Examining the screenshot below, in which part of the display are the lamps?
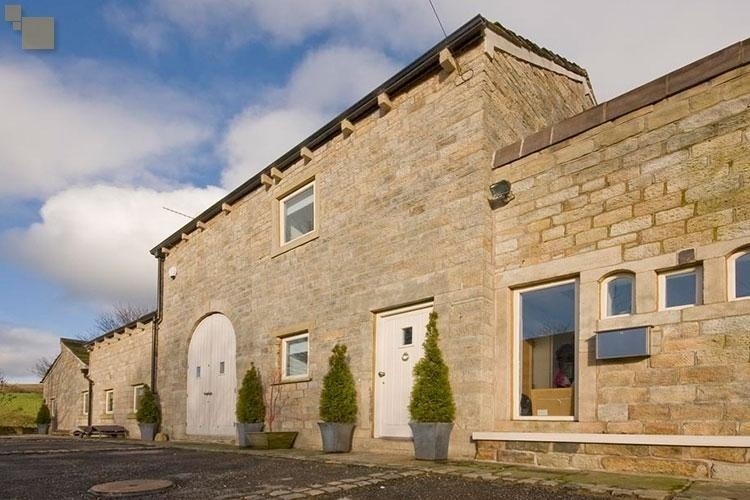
[490,180,515,204]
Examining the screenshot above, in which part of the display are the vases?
[245,431,299,449]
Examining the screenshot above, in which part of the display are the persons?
[551,361,572,388]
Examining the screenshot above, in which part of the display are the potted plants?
[135,384,160,442]
[235,362,266,448]
[34,399,52,435]
[317,343,358,452]
[409,311,457,460]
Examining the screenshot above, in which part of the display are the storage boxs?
[531,388,574,416]
